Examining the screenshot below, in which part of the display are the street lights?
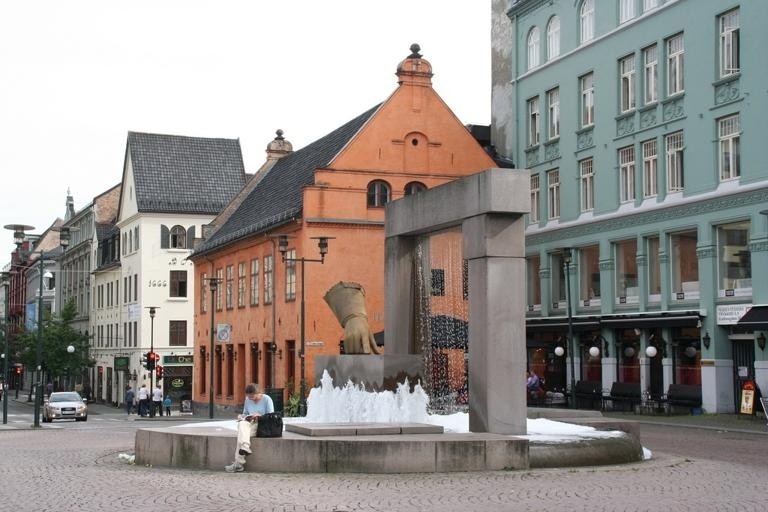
[553,245,583,410]
[4,222,80,426]
[268,232,338,417]
[1,353,7,393]
[145,305,161,416]
[203,277,226,419]
[0,270,20,426]
[13,361,24,398]
[36,363,46,407]
[66,342,75,392]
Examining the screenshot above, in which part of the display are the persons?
[46,381,92,402]
[0,379,5,401]
[528,368,540,388]
[134,387,141,416]
[527,371,531,385]
[222,381,275,473]
[125,387,135,415]
[140,384,149,417]
[322,281,382,354]
[151,385,163,417]
[163,395,171,417]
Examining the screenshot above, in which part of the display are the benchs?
[565,379,702,416]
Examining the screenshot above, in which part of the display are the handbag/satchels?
[257,413,283,437]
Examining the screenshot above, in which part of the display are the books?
[236,415,254,422]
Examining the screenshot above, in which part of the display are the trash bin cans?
[264,388,285,417]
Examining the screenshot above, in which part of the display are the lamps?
[702,332,711,349]
[553,334,609,357]
[757,332,766,351]
[645,336,667,359]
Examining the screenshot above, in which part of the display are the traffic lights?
[21,368,25,375]
[148,353,155,371]
[161,367,166,378]
[142,352,150,370]
[155,354,160,369]
[16,367,21,374]
[156,365,161,379]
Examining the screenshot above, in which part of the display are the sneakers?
[224,463,244,472]
[239,446,252,456]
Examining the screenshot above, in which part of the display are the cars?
[42,391,89,423]
